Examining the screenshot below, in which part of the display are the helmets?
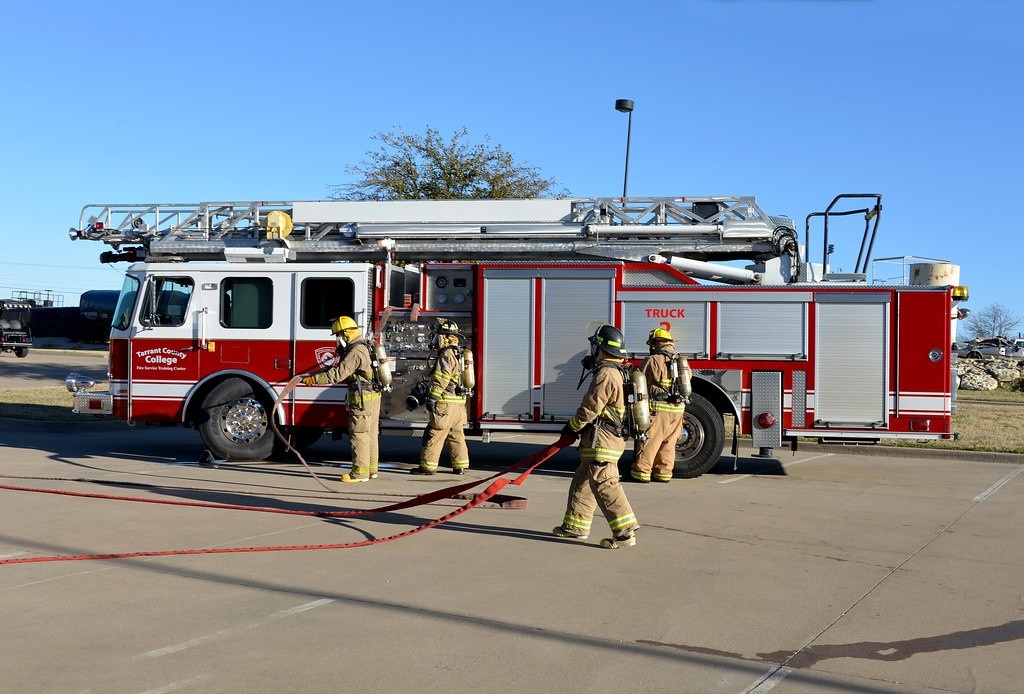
[646,329,676,346]
[590,325,626,358]
[434,321,466,340]
[330,316,358,336]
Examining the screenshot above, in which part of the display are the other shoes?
[341,474,370,483]
[599,527,635,549]
[410,465,436,475]
[650,473,670,483]
[553,527,588,539]
[621,473,649,483]
[452,468,464,475]
[369,474,378,479]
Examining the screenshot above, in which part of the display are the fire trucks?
[66,194,971,479]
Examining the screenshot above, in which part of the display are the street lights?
[615,99,634,226]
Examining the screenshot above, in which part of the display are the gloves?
[426,398,437,413]
[560,423,579,441]
[301,376,318,387]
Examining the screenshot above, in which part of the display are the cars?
[952,337,1024,359]
[0,310,33,358]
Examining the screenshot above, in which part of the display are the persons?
[405,318,469,475]
[553,320,640,549]
[302,316,382,483]
[621,328,693,483]
